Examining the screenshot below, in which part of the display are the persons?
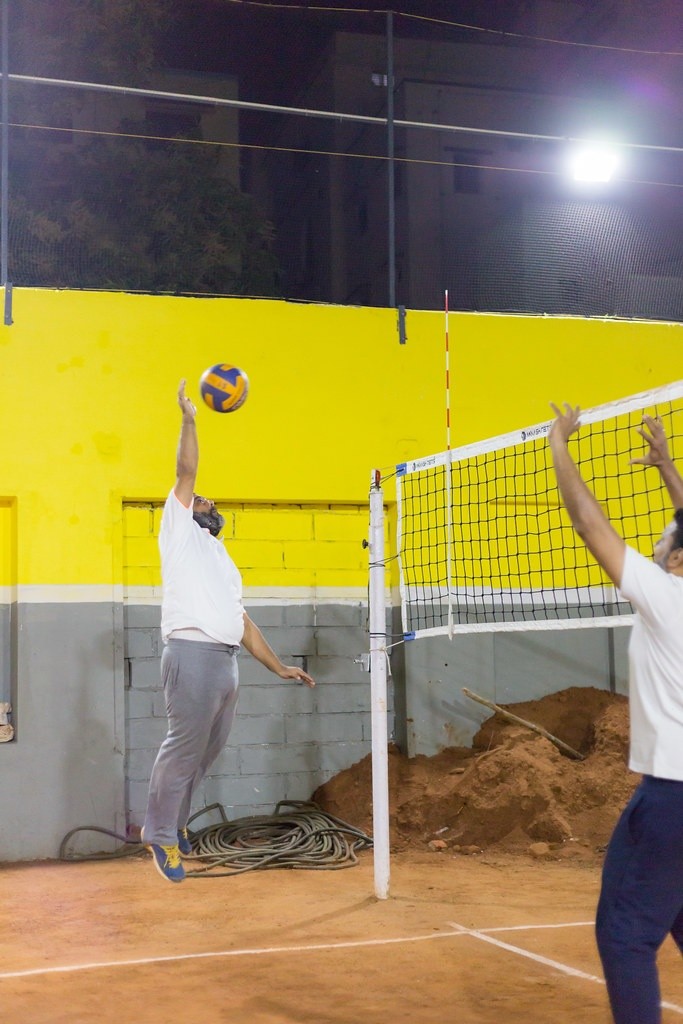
[139,379,318,884]
[548,400,683,1024]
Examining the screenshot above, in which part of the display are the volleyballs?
[200,363,250,413]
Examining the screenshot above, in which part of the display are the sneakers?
[141,826,185,883]
[176,827,192,857]
